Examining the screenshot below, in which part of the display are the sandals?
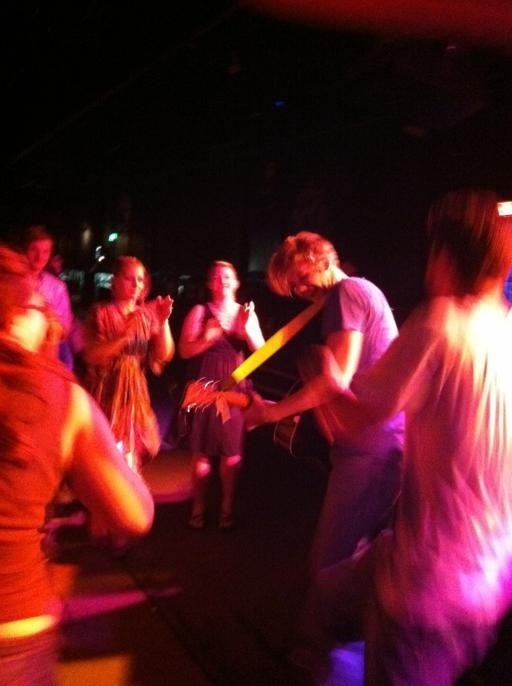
[217,510,233,528]
[188,511,206,529]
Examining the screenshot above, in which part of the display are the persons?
[0,248,156,686]
[240,230,407,673]
[307,197,510,686]
[0,225,88,378]
[81,256,176,549]
[178,260,267,530]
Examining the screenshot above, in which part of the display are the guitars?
[180,376,330,465]
